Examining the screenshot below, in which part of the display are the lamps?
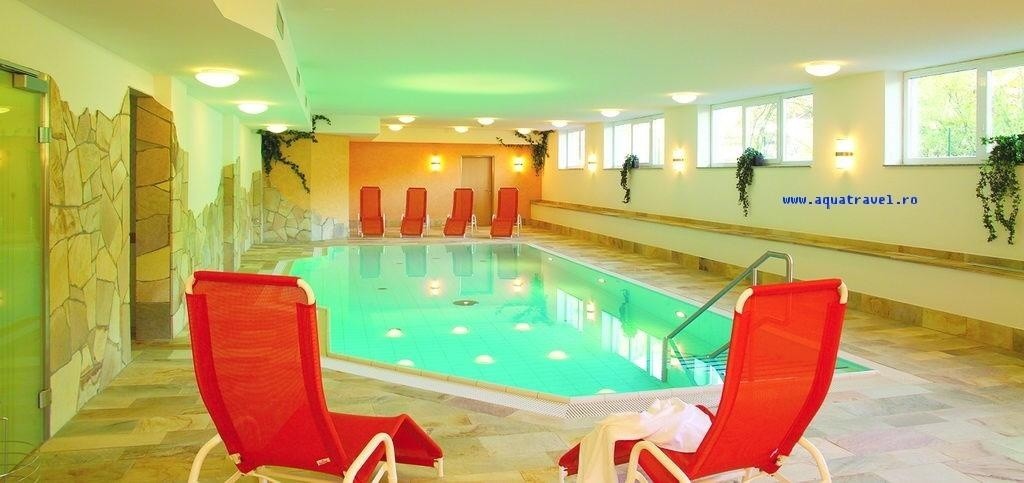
[833,134,857,169]
[512,157,523,173]
[587,153,596,171]
[671,146,686,172]
[428,156,440,172]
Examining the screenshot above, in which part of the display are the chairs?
[552,277,849,483]
[356,183,386,238]
[486,185,522,239]
[441,187,478,237]
[357,244,521,285]
[396,187,431,238]
[182,269,446,483]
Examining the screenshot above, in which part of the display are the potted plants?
[617,153,640,205]
[733,145,766,221]
